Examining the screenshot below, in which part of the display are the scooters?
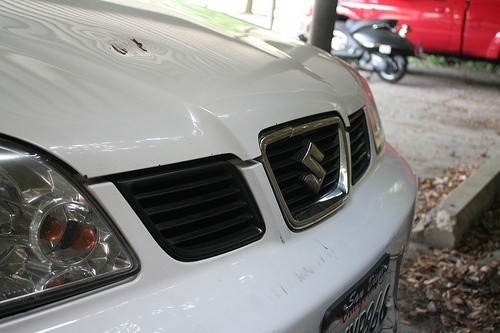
[301,15,414,82]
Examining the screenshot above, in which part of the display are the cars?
[305,0,500,82]
[0,1,416,333]
